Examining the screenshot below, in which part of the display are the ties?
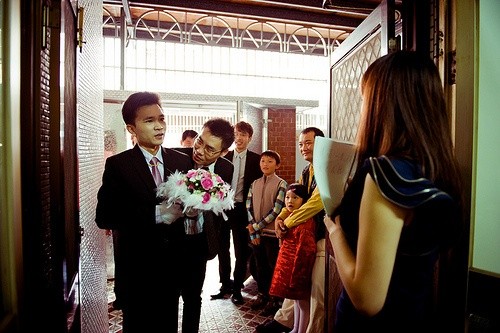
[230,155,241,194]
[150,158,166,189]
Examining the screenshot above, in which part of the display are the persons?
[94,92,201,333]
[180,129,199,149]
[255,126,327,333]
[323,49,462,333]
[170,118,234,333]
[245,150,289,317]
[268,183,317,333]
[210,121,265,305]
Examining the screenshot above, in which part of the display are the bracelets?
[328,228,343,239]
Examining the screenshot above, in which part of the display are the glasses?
[192,129,223,156]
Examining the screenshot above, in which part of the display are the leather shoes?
[232,292,243,304]
[211,287,231,299]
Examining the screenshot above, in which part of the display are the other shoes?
[263,301,282,313]
[248,293,268,308]
[255,319,291,333]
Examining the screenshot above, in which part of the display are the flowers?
[156,168,236,222]
